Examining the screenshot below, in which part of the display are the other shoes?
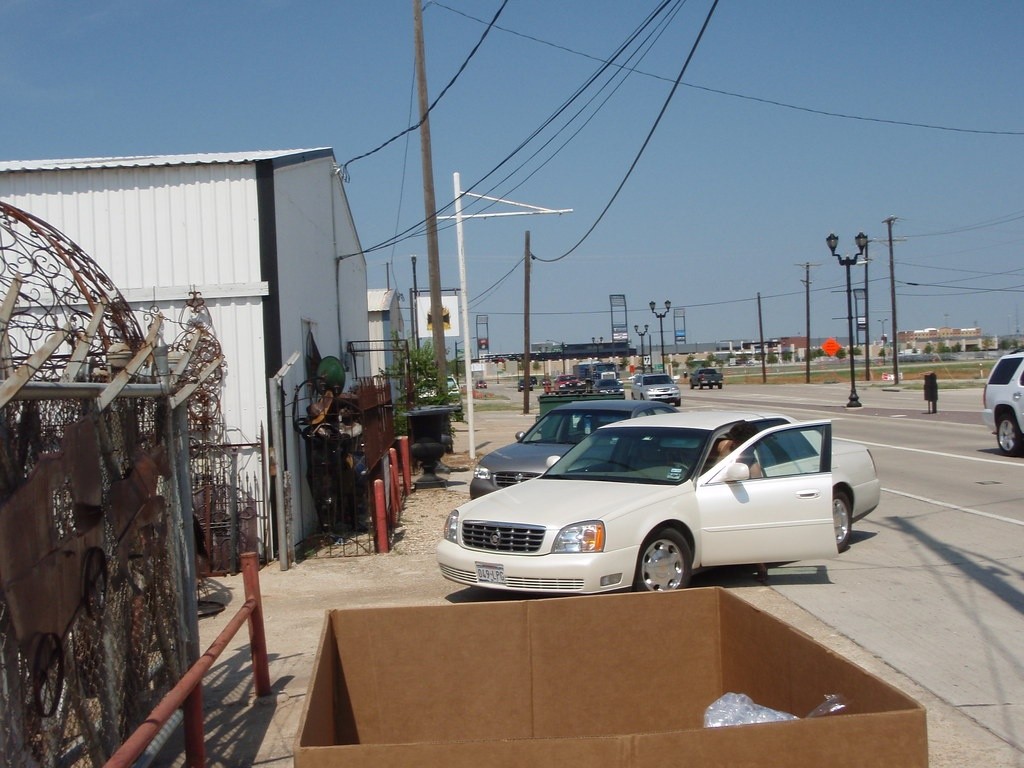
[756,567,768,582]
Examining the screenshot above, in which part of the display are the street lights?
[649,300,672,373]
[634,324,649,374]
[825,231,868,408]
[877,318,888,365]
[592,337,603,361]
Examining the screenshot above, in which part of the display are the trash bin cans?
[923,373,938,414]
[535,392,625,439]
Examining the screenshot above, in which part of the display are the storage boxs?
[292,587,928,768]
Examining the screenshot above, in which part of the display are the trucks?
[572,362,622,382]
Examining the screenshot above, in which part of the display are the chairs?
[643,448,685,471]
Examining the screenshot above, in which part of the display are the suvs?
[630,372,682,407]
[983,349,1024,457]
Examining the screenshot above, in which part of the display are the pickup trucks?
[689,368,724,389]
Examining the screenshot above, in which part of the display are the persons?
[705,423,765,582]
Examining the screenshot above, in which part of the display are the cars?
[475,380,487,390]
[539,379,551,388]
[469,399,681,500]
[530,377,537,386]
[554,371,625,395]
[417,376,460,397]
[436,409,880,595]
[518,379,534,392]
